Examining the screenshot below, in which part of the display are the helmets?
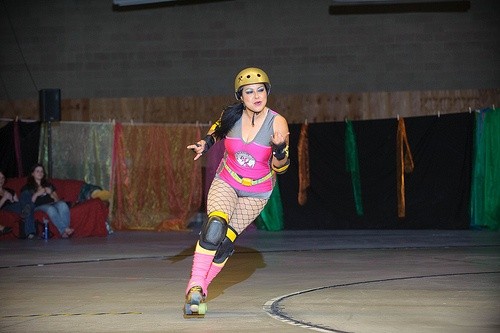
[234,67,271,101]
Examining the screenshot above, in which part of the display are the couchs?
[0,177,109,242]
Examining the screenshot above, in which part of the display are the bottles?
[105,222,113,234]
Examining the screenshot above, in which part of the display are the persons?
[184,66,291,315]
[0,171,37,239]
[21,163,74,238]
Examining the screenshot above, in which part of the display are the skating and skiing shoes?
[183,282,207,318]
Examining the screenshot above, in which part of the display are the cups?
[42,222,49,240]
